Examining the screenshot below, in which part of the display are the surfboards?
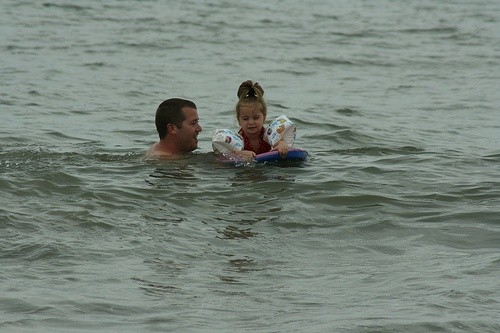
[214,147,309,164]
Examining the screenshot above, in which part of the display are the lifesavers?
[262,115,296,150]
[211,128,245,158]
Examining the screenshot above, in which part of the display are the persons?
[233,79,287,162]
[143,99,202,157]
